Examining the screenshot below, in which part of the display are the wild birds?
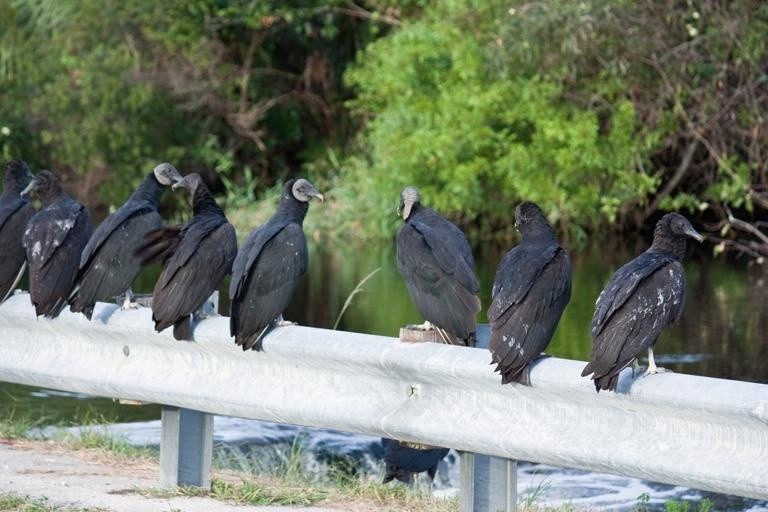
[227,176,326,353]
[18,168,94,322]
[0,157,38,307]
[378,434,451,489]
[132,169,238,343]
[64,159,186,322]
[393,186,485,347]
[580,208,709,393]
[483,199,574,386]
